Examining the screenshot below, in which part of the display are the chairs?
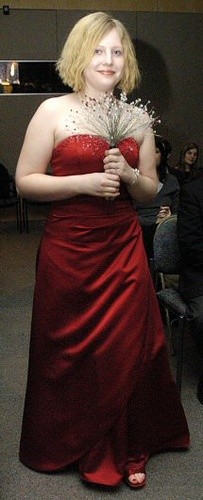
[0,163,22,234]
[153,214,195,400]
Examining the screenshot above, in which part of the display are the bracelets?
[127,168,140,186]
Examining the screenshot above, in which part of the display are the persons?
[15,12,203,490]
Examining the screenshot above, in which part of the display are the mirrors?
[0,59,75,95]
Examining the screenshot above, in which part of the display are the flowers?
[66,91,162,202]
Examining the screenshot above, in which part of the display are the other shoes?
[124,467,146,489]
